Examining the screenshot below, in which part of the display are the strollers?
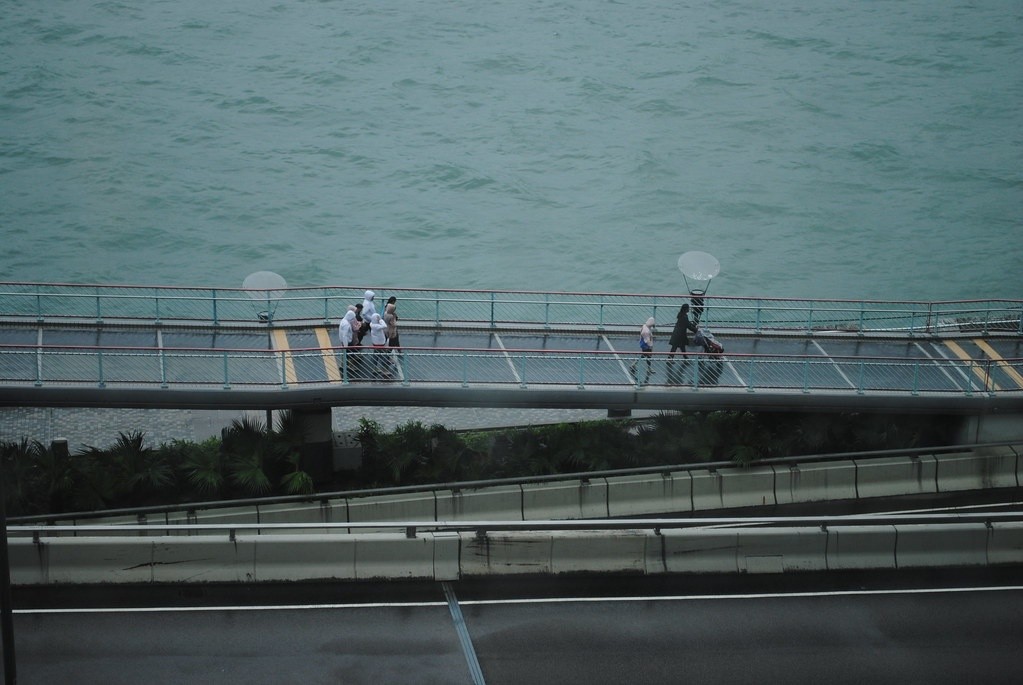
[693,322,724,370]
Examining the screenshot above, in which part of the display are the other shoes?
[382,371,392,376]
[668,360,675,363]
[684,360,695,364]
[630,366,638,371]
[646,369,656,373]
[373,369,383,373]
[348,366,358,371]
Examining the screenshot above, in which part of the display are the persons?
[666,304,698,364]
[338,304,368,373]
[370,313,392,377]
[630,317,656,374]
[381,296,403,358]
[360,290,377,333]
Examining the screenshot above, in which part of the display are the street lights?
[677,251,721,328]
[241,271,288,433]
